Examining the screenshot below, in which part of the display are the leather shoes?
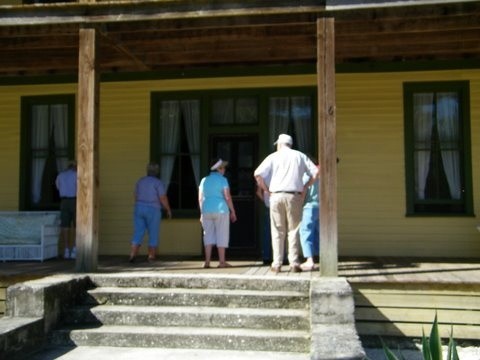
[271,264,303,273]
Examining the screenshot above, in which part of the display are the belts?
[275,190,301,194]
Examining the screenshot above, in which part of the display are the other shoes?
[123,254,158,263]
[64,247,76,259]
[300,262,314,268]
[201,259,233,269]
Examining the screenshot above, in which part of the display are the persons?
[129,163,172,258]
[198,159,238,269]
[256,182,273,265]
[55,160,79,258]
[253,133,319,272]
[299,153,319,269]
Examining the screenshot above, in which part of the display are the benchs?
[0,210,62,263]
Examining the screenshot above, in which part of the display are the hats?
[274,133,293,146]
[210,159,228,171]
[64,161,76,169]
